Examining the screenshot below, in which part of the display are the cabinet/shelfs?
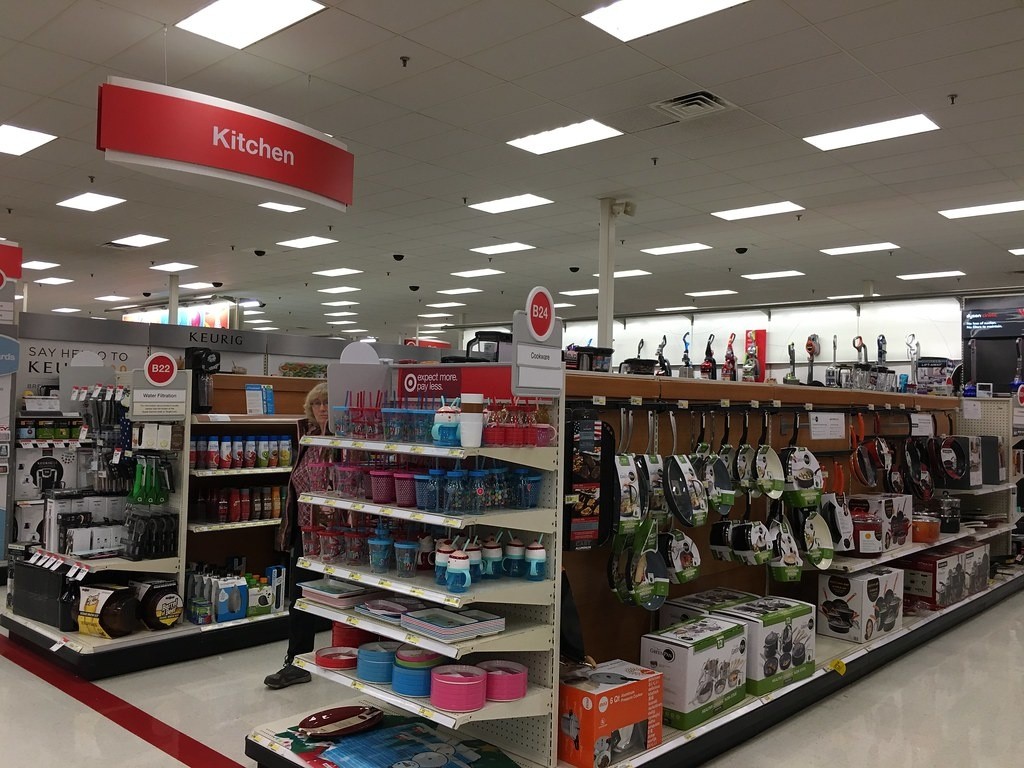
[0,372,130,586]
[0,373,329,683]
[245,360,1024,768]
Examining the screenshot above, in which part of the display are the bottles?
[189,434,294,522]
[242,573,270,589]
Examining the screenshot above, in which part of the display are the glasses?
[311,401,328,406]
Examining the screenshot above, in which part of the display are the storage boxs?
[640,586,816,730]
[14,418,127,557]
[558,660,664,768]
[818,567,903,643]
[844,492,913,553]
[882,541,990,611]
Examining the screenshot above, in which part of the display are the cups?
[300,405,558,592]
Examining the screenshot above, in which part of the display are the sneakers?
[264,655,311,689]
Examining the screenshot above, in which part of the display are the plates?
[296,579,506,643]
[298,706,383,736]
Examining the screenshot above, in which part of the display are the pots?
[608,411,1024,609]
[761,624,811,677]
[682,592,789,614]
[687,655,748,705]
[560,568,597,670]
[820,500,992,633]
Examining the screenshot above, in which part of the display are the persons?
[263,381,340,690]
